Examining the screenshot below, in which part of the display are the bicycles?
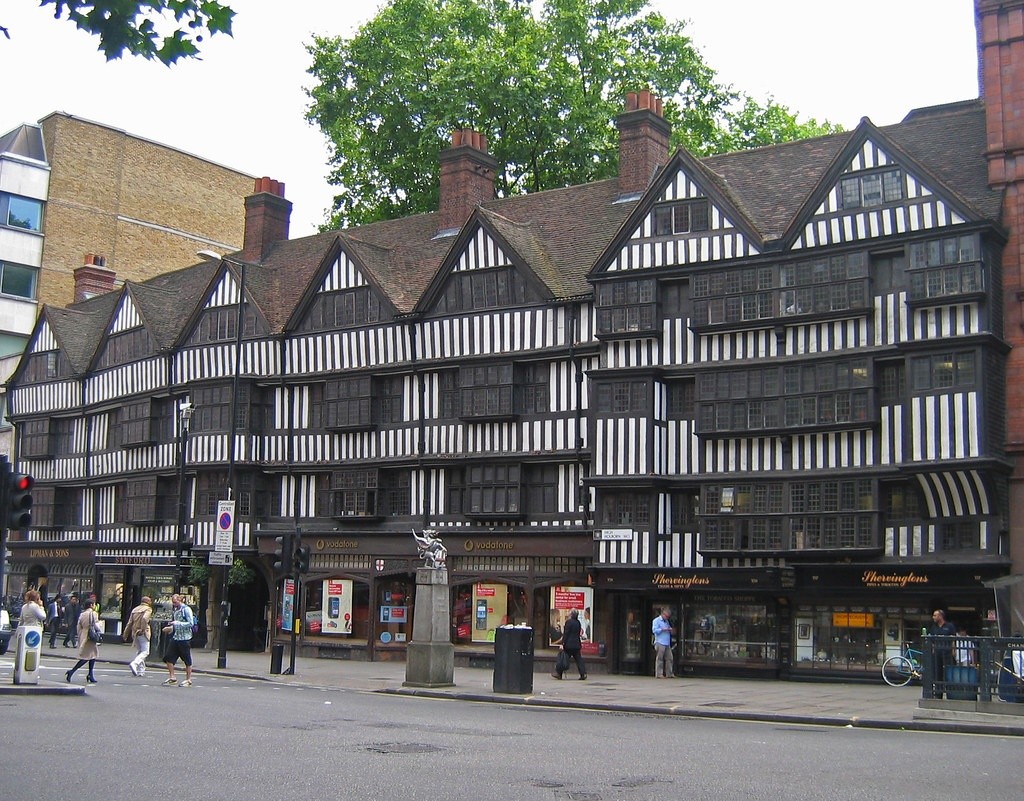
[881,640,925,688]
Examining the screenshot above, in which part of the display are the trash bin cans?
[144,620,170,662]
[492,627,535,694]
[270,644,284,674]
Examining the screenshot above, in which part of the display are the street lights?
[194,247,248,670]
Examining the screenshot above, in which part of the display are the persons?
[65,598,100,682]
[162,593,194,687]
[651,606,678,679]
[16,590,46,637]
[928,609,956,700]
[953,627,976,667]
[46,594,102,649]
[123,596,152,677]
[551,608,587,680]
[1004,632,1024,684]
[550,609,564,646]
[583,607,590,640]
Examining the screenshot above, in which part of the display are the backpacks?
[181,605,198,633]
[556,650,569,673]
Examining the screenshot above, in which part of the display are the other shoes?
[73,645,78,649]
[50,644,57,649]
[130,662,138,677]
[139,672,145,676]
[551,672,562,680]
[667,675,677,679]
[63,642,70,648]
[656,675,665,679]
[162,677,178,686]
[578,672,587,681]
[179,680,192,687]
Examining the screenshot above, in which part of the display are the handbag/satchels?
[89,612,100,642]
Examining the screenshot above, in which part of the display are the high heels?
[65,670,74,683]
[87,674,98,683]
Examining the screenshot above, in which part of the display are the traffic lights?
[293,547,309,574]
[4,471,36,531]
[274,534,296,573]
